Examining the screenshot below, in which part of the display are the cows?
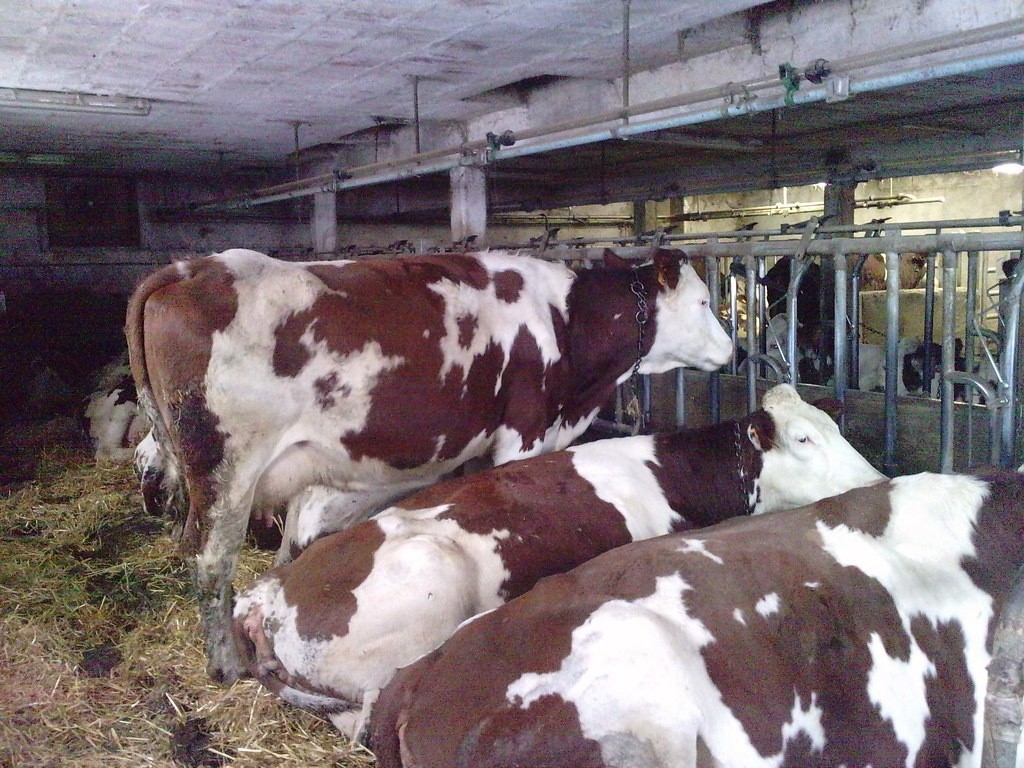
[77,250,1024,768]
